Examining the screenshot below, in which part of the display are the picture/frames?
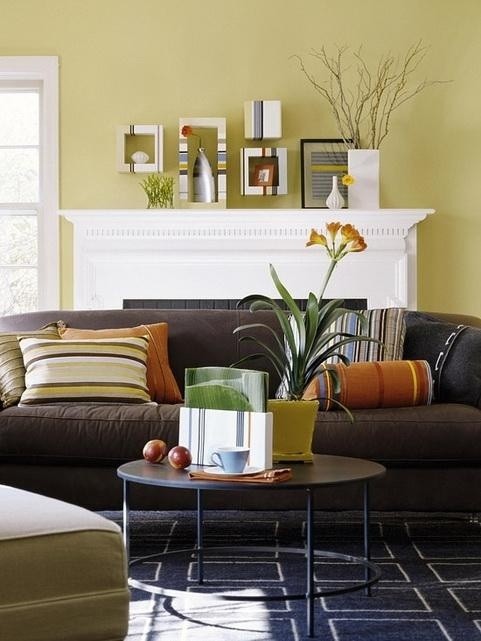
[300,137,358,209]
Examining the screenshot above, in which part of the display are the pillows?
[0,321,67,409]
[403,311,481,408]
[274,307,410,401]
[16,335,151,404]
[56,320,185,405]
[301,359,434,409]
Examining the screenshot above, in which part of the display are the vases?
[347,148,381,211]
[261,397,322,464]
[325,176,346,209]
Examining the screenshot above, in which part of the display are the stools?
[0,482,131,641]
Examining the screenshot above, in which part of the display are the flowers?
[228,217,387,426]
[335,171,357,185]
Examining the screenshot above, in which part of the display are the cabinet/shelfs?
[113,100,289,207]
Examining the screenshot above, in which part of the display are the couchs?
[0,307,481,514]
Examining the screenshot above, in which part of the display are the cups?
[210,447,251,473]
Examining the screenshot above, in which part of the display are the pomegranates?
[142,439,167,464]
[167,447,192,469]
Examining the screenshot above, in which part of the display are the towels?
[186,465,294,485]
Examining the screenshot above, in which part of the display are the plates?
[203,466,266,476]
